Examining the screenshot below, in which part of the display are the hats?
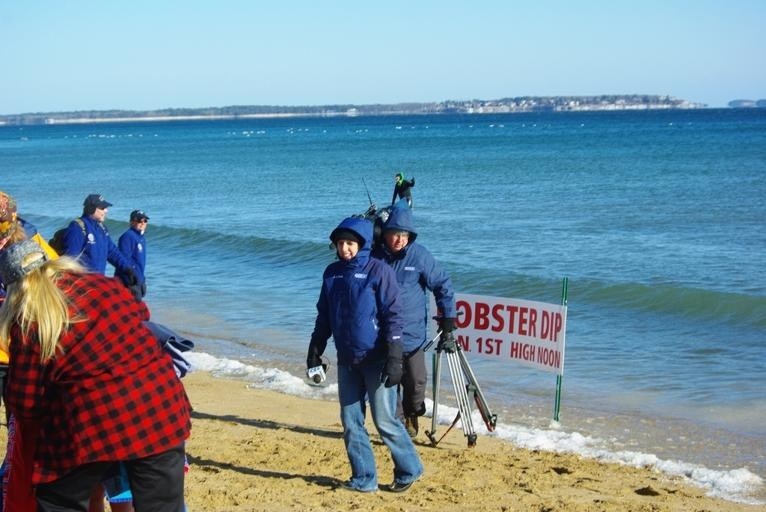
[332,232,361,244]
[0,191,17,243]
[0,240,48,291]
[84,195,112,210]
[131,211,150,220]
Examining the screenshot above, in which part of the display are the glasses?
[137,220,147,223]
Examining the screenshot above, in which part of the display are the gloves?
[438,317,457,333]
[381,358,403,387]
[307,354,327,375]
[126,270,137,286]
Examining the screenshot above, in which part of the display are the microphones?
[306,363,328,386]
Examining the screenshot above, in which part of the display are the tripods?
[423,316,498,448]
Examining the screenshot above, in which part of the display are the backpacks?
[49,218,86,260]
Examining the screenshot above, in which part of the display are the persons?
[0,241,193,512]
[1,190,59,432]
[369,199,456,438]
[306,219,424,492]
[391,172,415,209]
[62,193,138,287]
[88,473,134,512]
[114,210,148,302]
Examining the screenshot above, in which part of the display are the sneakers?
[387,479,412,491]
[406,416,418,437]
[340,481,356,490]
